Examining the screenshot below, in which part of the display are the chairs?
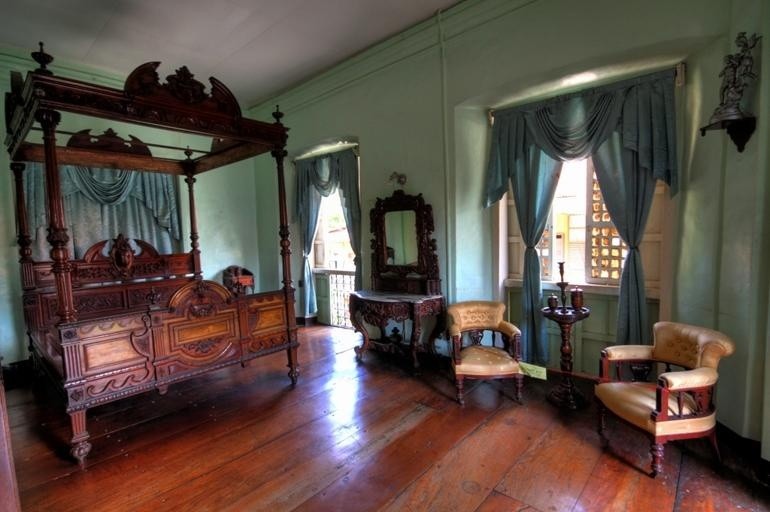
[443,300,525,408]
[595,320,737,477]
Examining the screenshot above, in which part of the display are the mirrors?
[371,188,439,281]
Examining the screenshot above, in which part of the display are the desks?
[542,307,590,412]
[349,289,444,368]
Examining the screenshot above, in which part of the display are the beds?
[27,235,300,469]
